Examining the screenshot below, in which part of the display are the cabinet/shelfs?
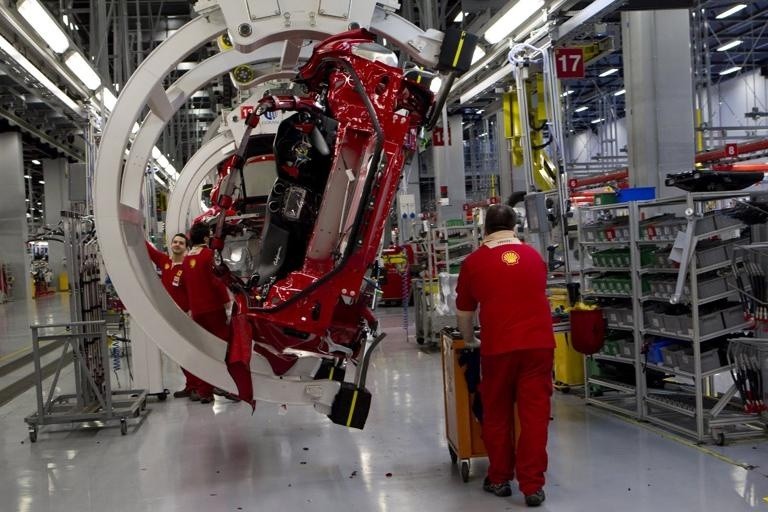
[432,215,479,280]
[577,191,761,445]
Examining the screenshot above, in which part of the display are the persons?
[145,223,242,403]
[454,203,556,507]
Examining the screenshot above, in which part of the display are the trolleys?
[434,319,527,484]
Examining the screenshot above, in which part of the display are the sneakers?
[482,473,512,497]
[173,387,215,404]
[523,486,545,508]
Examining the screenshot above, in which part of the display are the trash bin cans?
[552,321,591,393]
